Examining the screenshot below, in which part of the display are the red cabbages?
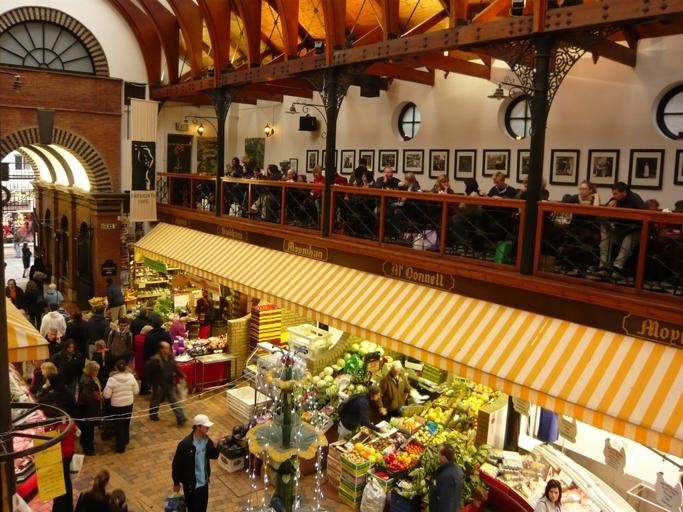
[218,408,272,450]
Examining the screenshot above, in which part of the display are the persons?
[4,213,34,278]
[429,445,464,512]
[4,259,232,455]
[171,414,223,512]
[337,386,385,440]
[373,362,415,424]
[271,459,296,512]
[533,479,562,512]
[73,470,129,512]
[194,156,683,295]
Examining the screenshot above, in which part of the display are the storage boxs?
[326,360,493,512]
[218,444,245,473]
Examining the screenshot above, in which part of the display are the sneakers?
[150,416,160,421]
[177,418,188,426]
[83,451,95,456]
[116,446,124,452]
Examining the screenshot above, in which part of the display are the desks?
[192,352,240,400]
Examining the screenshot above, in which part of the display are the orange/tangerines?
[404,443,425,455]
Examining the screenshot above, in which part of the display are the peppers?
[384,452,418,472]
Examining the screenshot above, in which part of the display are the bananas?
[432,430,458,445]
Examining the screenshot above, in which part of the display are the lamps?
[487,83,534,135]
[184,115,217,132]
[265,124,274,138]
[286,102,328,138]
[0,71,21,92]
[197,124,204,136]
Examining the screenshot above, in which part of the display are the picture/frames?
[289,149,531,183]
[549,148,683,191]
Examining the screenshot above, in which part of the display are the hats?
[193,414,214,427]
[95,340,109,352]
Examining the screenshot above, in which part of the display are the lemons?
[462,383,502,416]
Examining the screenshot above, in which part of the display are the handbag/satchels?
[163,492,186,511]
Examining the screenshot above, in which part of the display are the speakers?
[299,116,316,130]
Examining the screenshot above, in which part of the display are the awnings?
[134,222,683,458]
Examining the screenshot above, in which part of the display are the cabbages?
[311,340,385,390]
